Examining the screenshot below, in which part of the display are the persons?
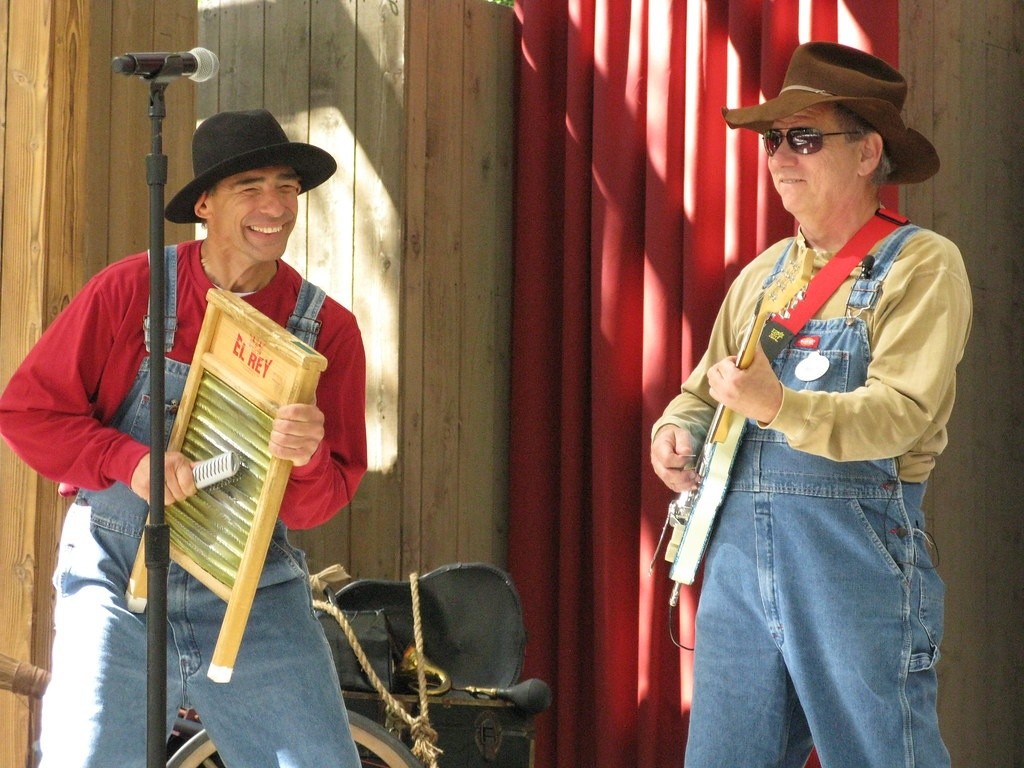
[1,108,367,768]
[651,40,977,768]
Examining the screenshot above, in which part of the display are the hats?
[721,41,940,186]
[164,108,338,224]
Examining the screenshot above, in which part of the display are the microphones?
[113,47,219,83]
[862,255,875,274]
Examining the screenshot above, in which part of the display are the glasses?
[762,127,869,155]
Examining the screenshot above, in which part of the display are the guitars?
[661,244,817,586]
